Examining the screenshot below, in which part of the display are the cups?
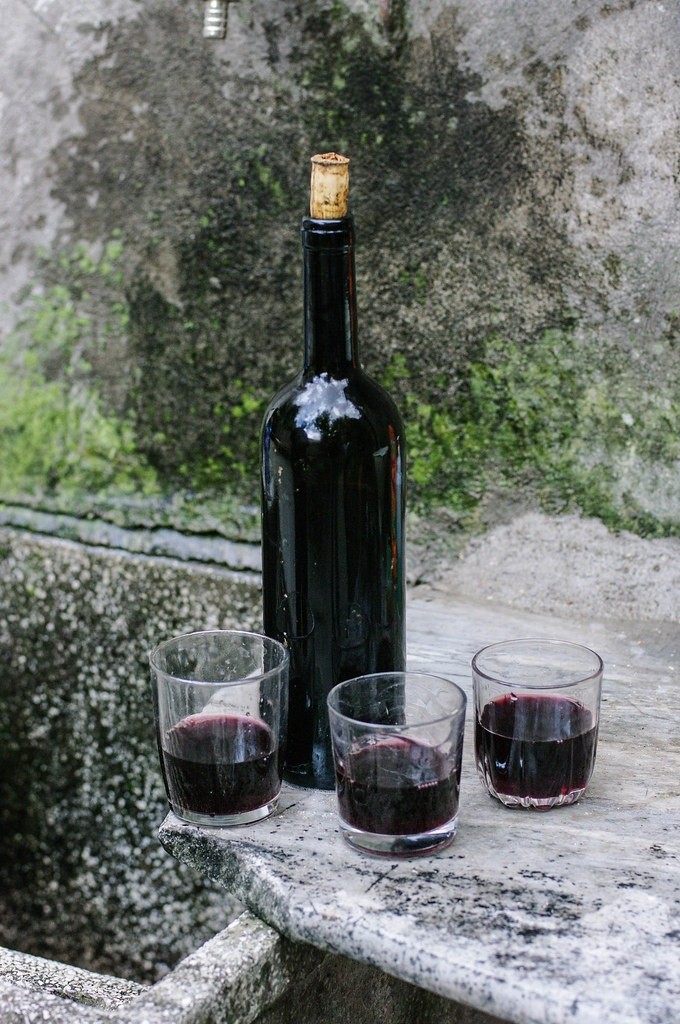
[146,630,289,827]
[471,641,604,811]
[325,671,467,859]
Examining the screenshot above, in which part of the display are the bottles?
[260,153,407,789]
